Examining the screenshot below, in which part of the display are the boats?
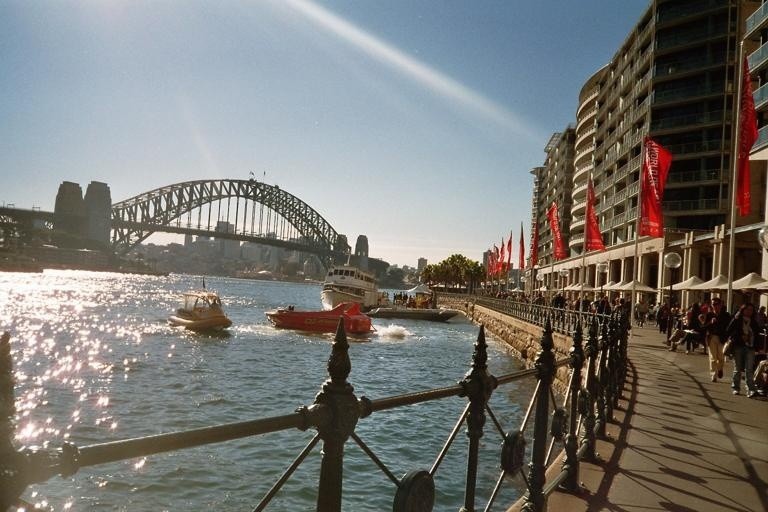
[264,301,377,334]
[321,254,393,312]
[168,276,233,334]
[364,305,459,322]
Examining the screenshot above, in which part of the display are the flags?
[485,170,605,340]
[635,133,673,239]
[733,44,760,215]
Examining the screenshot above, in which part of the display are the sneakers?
[732,390,739,395]
[747,392,756,398]
[717,369,723,379]
[712,375,717,382]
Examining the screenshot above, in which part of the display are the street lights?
[486,281,491,296]
[758,225,768,255]
[492,280,499,297]
[536,273,544,305]
[500,279,506,298]
[596,261,609,313]
[465,281,468,294]
[508,277,514,299]
[663,252,682,346]
[558,267,570,309]
[481,282,485,296]
[520,276,527,301]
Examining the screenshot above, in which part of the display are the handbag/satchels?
[722,340,733,355]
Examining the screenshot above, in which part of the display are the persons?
[391,292,438,310]
[210,298,219,309]
[287,305,294,313]
[481,271,767,396]
